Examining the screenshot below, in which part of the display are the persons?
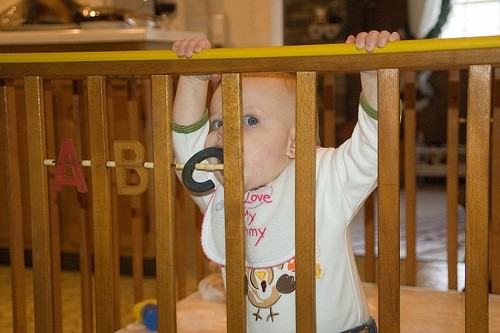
[171,30,401,333]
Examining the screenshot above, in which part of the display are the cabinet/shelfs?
[0,29,208,261]
